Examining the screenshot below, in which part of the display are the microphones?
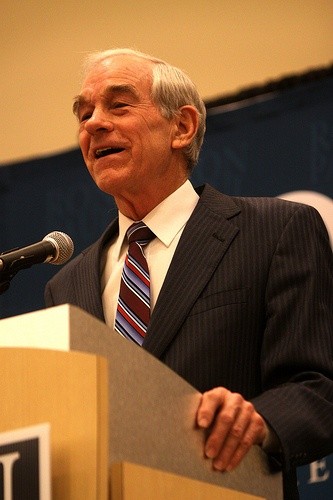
[0,231,74,273]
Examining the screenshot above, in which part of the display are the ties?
[113,221,157,346]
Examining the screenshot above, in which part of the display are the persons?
[44,48,333,500]
